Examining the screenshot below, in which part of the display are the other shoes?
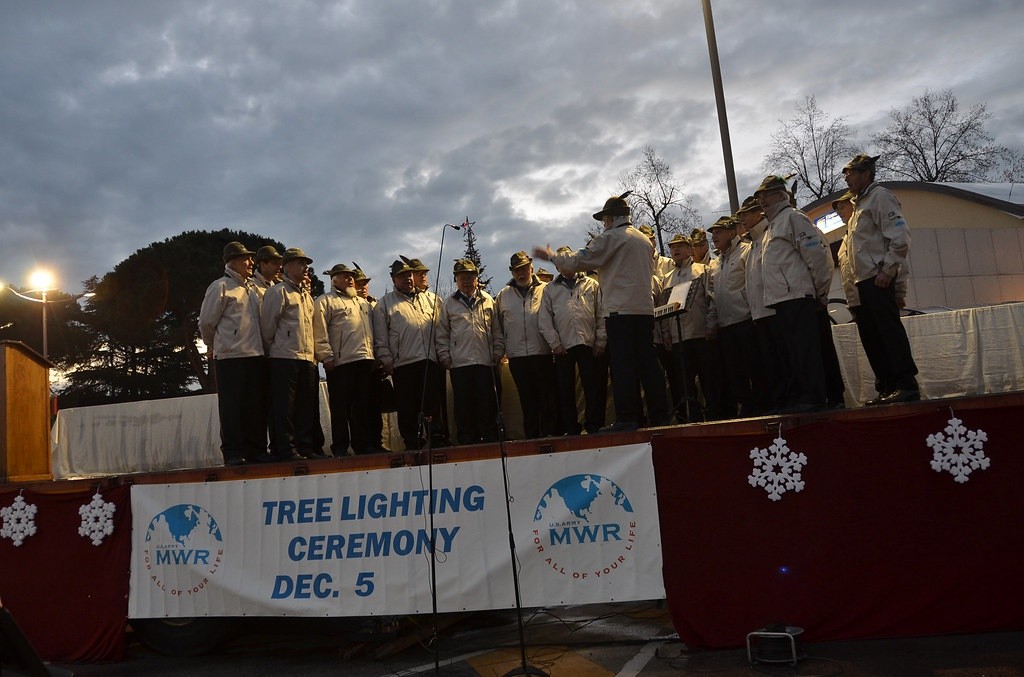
[404,432,506,451]
[226,441,393,463]
[598,419,641,431]
[865,387,920,405]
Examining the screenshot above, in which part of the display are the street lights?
[0,268,95,361]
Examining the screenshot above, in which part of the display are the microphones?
[446,223,460,230]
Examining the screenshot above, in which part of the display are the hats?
[752,174,787,196]
[832,192,855,210]
[736,196,761,215]
[842,155,881,173]
[388,260,414,274]
[668,233,690,245]
[689,229,707,242]
[350,269,372,283]
[509,252,532,269]
[281,248,312,263]
[254,246,283,262]
[327,264,356,275]
[639,225,656,239]
[556,246,573,253]
[405,259,430,272]
[454,259,479,273]
[707,216,733,232]
[535,268,554,279]
[223,242,256,263]
[593,197,631,221]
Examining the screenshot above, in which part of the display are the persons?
[533,196,672,433]
[638,154,920,424]
[200,242,609,464]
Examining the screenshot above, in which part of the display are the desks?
[49,381,331,481]
[830,302,1024,408]
